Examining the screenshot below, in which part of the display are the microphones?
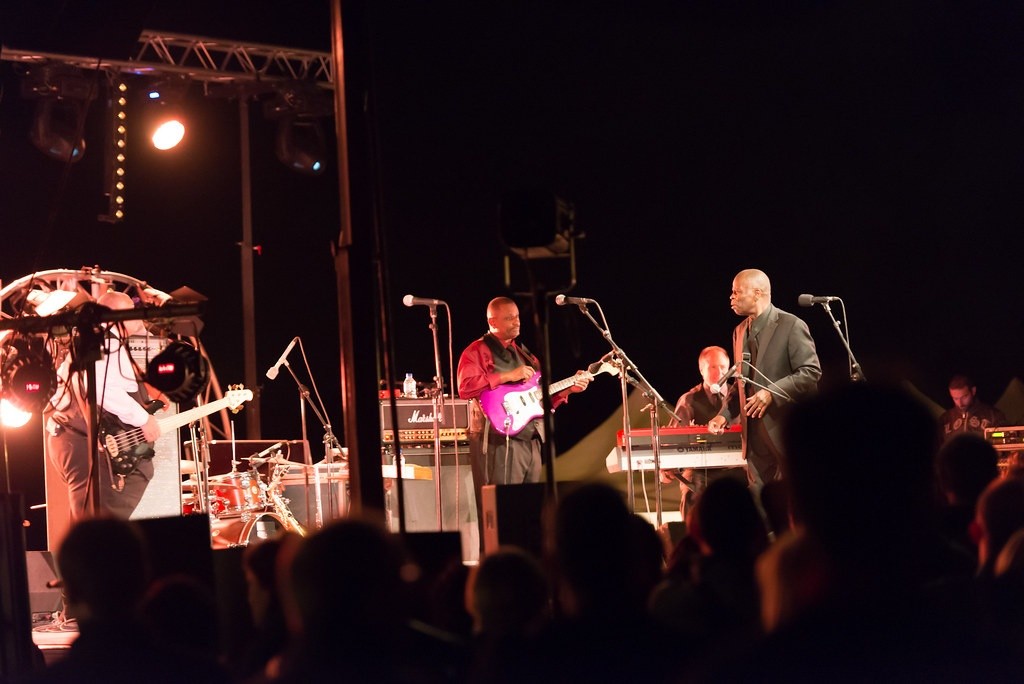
[265,338,297,380]
[588,351,616,374]
[798,294,838,307]
[710,361,741,394]
[403,295,445,307]
[555,294,594,305]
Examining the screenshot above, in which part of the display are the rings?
[757,408,761,410]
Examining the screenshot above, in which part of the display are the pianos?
[606,423,748,531]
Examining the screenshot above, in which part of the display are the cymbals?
[181,459,210,475]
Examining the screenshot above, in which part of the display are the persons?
[935,374,1012,458]
[42,291,161,523]
[457,296,595,556]
[30,378,1024,684]
[659,269,823,542]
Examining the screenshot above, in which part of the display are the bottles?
[393,449,405,466]
[404,373,416,397]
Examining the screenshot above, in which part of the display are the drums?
[210,471,265,513]
[211,511,290,549]
[179,492,214,514]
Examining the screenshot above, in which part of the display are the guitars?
[477,350,623,436]
[97,383,254,477]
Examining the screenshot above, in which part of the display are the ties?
[507,344,522,366]
[715,391,722,411]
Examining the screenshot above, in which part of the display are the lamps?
[143,104,185,150]
